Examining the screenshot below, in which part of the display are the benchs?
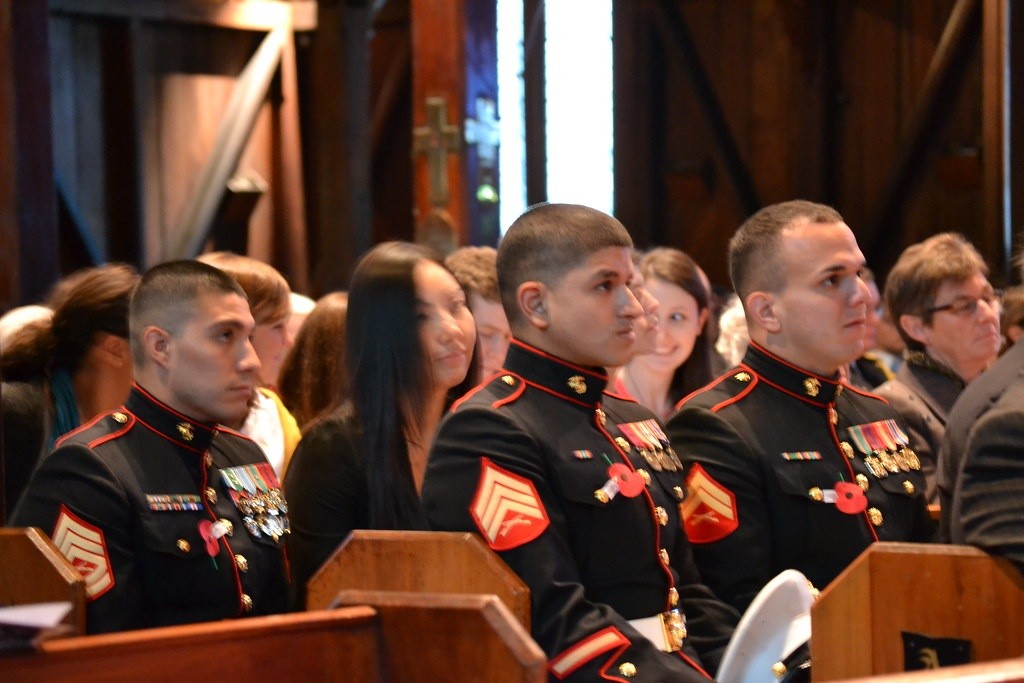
[1,525,86,635]
[0,590,547,683]
[306,530,530,638]
[810,544,1024,683]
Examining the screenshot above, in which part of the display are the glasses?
[912,283,1006,317]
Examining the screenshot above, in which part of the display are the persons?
[0,232,1023,520]
[661,201,971,683]
[937,332,1024,580]
[421,205,741,683]
[0,259,300,656]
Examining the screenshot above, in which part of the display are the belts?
[626,607,687,653]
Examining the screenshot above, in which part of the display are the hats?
[715,568,821,683]
